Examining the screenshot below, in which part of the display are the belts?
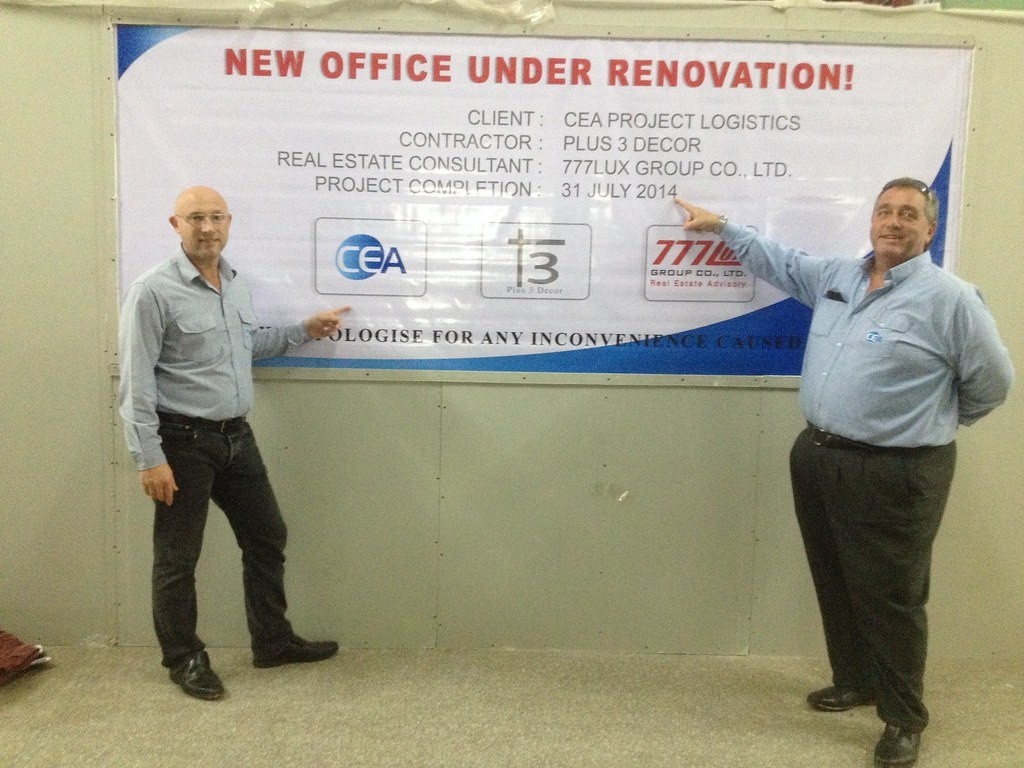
[806,421,921,457]
[156,412,247,433]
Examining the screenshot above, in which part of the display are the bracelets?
[714,214,729,235]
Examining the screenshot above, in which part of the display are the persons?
[672,175,1014,768]
[114,186,354,701]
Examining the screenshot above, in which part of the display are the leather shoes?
[253,634,338,668]
[808,685,875,712]
[170,650,224,699]
[874,721,921,768]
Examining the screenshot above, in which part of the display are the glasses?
[176,213,231,226]
[883,177,932,199]
